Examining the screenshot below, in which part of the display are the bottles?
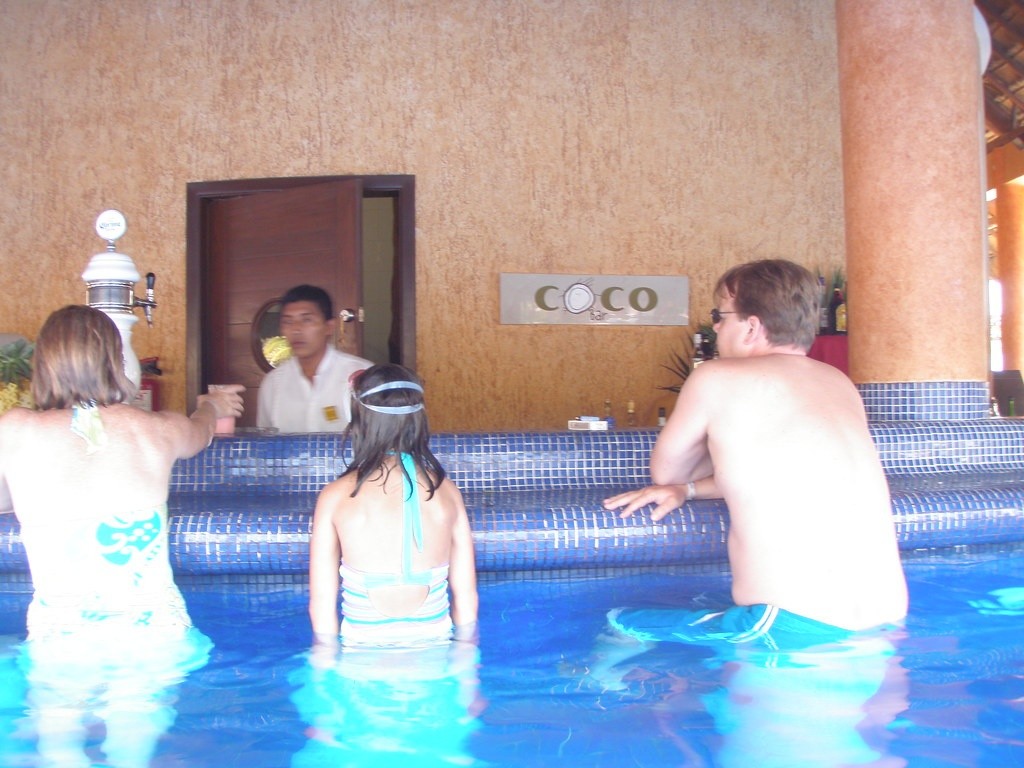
[601,400,615,429]
[625,399,640,426]
[656,408,667,426]
[830,287,847,334]
[1007,397,1015,416]
[690,333,719,368]
[817,277,829,334]
[992,399,999,416]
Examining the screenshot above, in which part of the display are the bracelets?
[687,481,697,502]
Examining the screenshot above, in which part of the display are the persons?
[306,364,481,656]
[600,259,910,660]
[0,303,247,650]
[257,284,375,432]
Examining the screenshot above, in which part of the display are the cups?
[209,384,235,437]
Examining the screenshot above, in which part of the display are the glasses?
[710,309,742,323]
